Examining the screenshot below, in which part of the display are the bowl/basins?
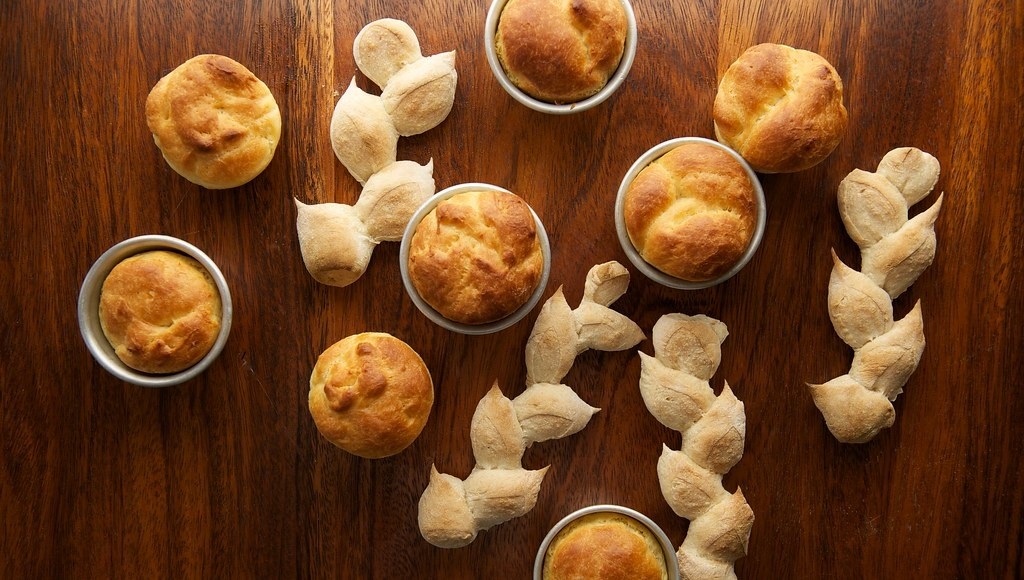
[483,0,636,114]
[614,137,766,287]
[77,234,233,387]
[533,504,680,580]
[400,184,554,335]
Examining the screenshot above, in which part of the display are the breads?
[97,0,946,580]
